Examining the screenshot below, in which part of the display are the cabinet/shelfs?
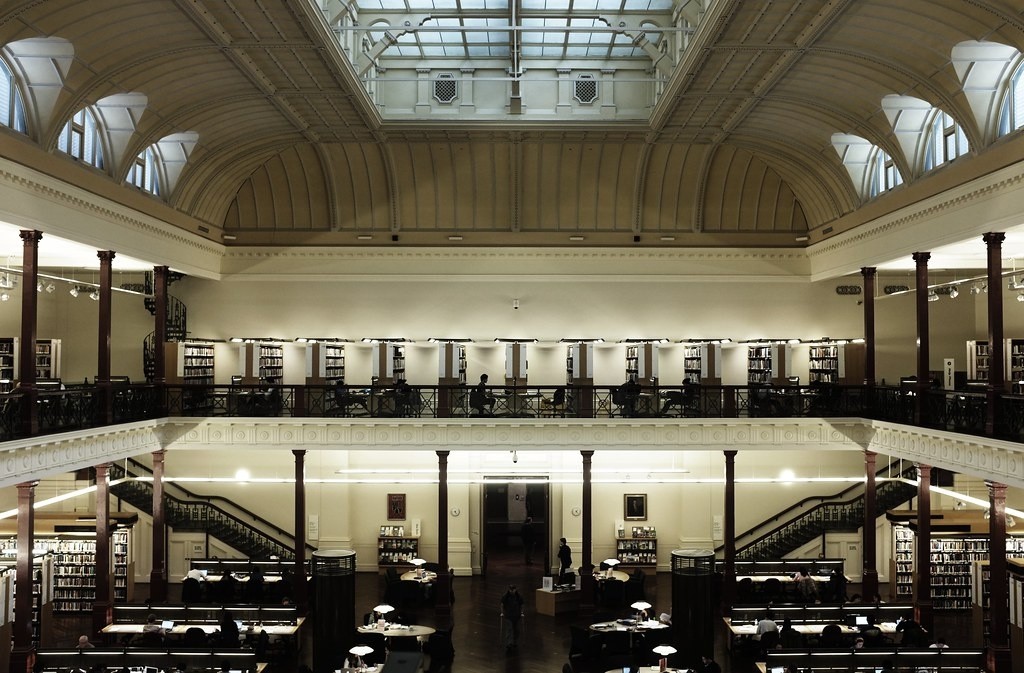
[164,339,868,418]
[616,538,659,575]
[0,337,62,394]
[891,525,1024,610]
[1007,338,1024,382]
[967,339,990,381]
[0,511,139,666]
[374,537,420,576]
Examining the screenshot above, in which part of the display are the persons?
[613,379,635,415]
[520,517,547,566]
[475,373,496,416]
[699,655,721,673]
[661,377,695,414]
[558,537,572,587]
[261,378,281,417]
[501,584,525,650]
[735,567,949,673]
[336,380,367,409]
[75,570,287,673]
[396,379,415,418]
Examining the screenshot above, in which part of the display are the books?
[896,527,1024,610]
[0,533,127,644]
[2,339,1024,406]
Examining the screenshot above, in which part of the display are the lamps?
[0,253,100,301]
[603,559,620,577]
[349,644,374,666]
[653,645,677,673]
[630,601,652,622]
[409,559,427,569]
[372,603,395,619]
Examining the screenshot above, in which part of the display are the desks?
[335,568,687,672]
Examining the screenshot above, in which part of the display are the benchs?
[32,557,328,672]
[718,600,985,673]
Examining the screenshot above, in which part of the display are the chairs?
[490,392,542,417]
[343,391,392,416]
[469,389,487,411]
[779,391,815,418]
[925,258,1024,303]
[543,389,565,412]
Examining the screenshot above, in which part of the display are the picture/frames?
[625,493,648,521]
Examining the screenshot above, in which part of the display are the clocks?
[451,508,461,516]
[572,507,581,516]
